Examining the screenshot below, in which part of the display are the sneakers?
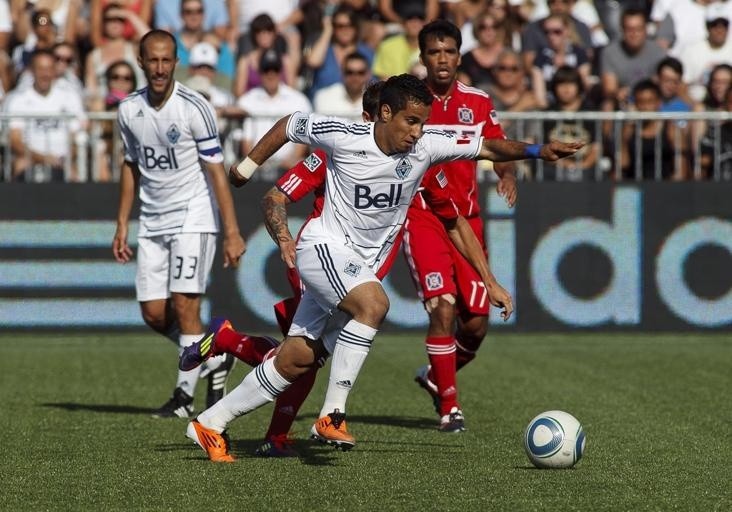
[413,364,466,433]
[153,318,356,461]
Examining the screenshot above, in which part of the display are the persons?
[111,28,249,419]
[184,73,587,462]
[0,0,732,181]
[401,18,518,432]
[176,80,515,458]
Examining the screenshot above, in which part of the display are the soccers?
[524,410,586,468]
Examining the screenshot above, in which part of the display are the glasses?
[183,6,201,15]
[344,67,366,77]
[57,54,72,66]
[335,22,352,30]
[111,74,132,82]
[479,23,563,73]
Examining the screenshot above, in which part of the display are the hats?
[189,42,220,68]
[708,1,730,23]
[258,49,284,68]
